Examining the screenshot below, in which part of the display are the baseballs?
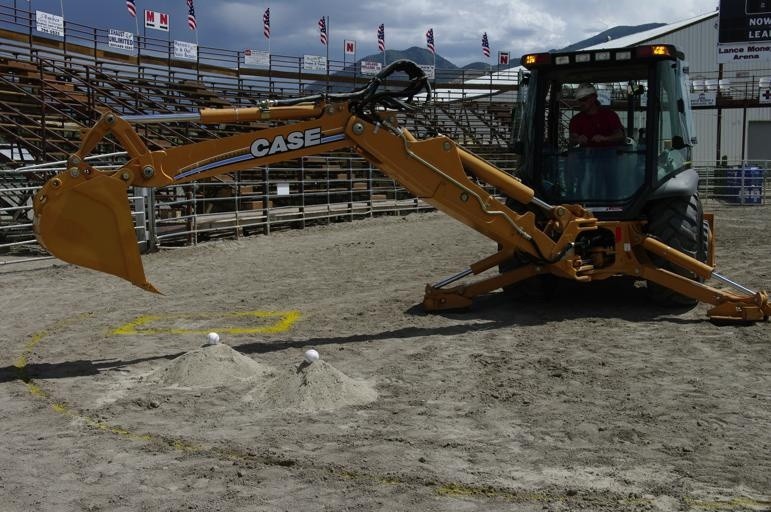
[207,332,219,345]
[304,351,318,366]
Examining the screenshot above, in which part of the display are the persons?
[564,81,627,203]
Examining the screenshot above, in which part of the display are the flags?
[377,23,385,54]
[127,0,137,19]
[263,7,271,40]
[426,28,436,56]
[318,16,328,46]
[481,31,490,58]
[187,0,198,32]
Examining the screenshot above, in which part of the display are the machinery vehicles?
[30,41,771,327]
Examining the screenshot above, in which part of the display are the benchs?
[0,44,517,252]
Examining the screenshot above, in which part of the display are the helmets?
[575,83,596,101]
